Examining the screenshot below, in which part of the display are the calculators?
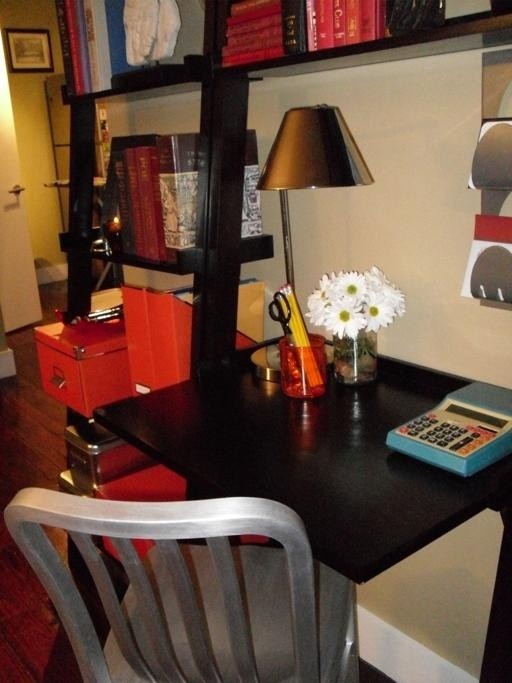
[385,381,512,478]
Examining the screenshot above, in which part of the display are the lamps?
[245,101,380,387]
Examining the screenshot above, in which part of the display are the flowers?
[306,267,411,364]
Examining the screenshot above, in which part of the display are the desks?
[93,333,512,683]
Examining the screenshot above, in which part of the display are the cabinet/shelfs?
[41,71,119,280]
[51,0,239,617]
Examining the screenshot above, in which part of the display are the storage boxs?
[56,468,93,496]
[91,456,274,563]
[33,312,125,418]
[64,420,157,486]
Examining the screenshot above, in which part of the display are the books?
[99,127,263,264]
[51,0,140,98]
[218,0,389,66]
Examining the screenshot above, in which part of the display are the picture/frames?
[4,27,54,74]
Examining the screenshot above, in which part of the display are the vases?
[331,330,377,383]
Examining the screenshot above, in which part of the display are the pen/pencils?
[277,283,324,387]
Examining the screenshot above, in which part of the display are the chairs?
[4,486,360,683]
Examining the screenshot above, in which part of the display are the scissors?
[269,292,295,346]
